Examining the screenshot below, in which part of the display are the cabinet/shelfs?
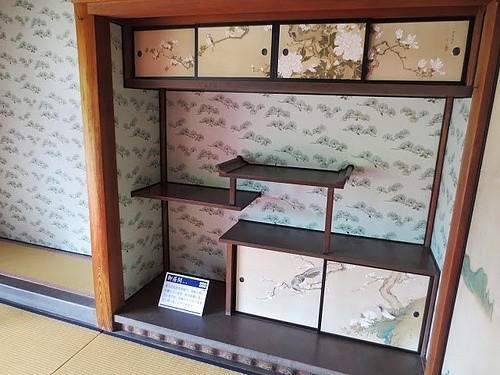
[109,6,486,374]
